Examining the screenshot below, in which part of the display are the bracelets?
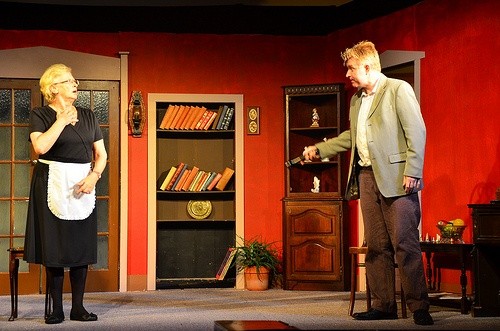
[93,170,101,178]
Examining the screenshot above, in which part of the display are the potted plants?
[231,232,280,291]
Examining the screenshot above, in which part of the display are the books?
[160,163,234,191]
[216,248,236,280]
[159,105,234,130]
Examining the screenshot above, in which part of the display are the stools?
[6,248,51,322]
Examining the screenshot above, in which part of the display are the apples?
[437,220,447,226]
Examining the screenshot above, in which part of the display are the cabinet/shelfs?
[281,83,347,292]
[467,204,500,317]
[147,93,245,290]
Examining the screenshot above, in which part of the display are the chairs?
[349,237,407,319]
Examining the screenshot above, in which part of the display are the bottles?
[419,233,441,244]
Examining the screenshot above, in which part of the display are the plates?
[187,200,212,220]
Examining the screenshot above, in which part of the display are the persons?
[312,109,319,125]
[300,41,434,325]
[22,63,107,324]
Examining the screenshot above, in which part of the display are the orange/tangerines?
[453,219,464,226]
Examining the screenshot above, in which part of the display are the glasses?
[52,79,80,86]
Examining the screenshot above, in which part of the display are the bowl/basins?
[437,225,466,244]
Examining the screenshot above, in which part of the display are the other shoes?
[70,308,98,321]
[413,310,434,325]
[353,307,399,320]
[45,311,65,324]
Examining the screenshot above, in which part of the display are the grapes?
[444,224,457,231]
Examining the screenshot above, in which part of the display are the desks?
[419,242,473,315]
